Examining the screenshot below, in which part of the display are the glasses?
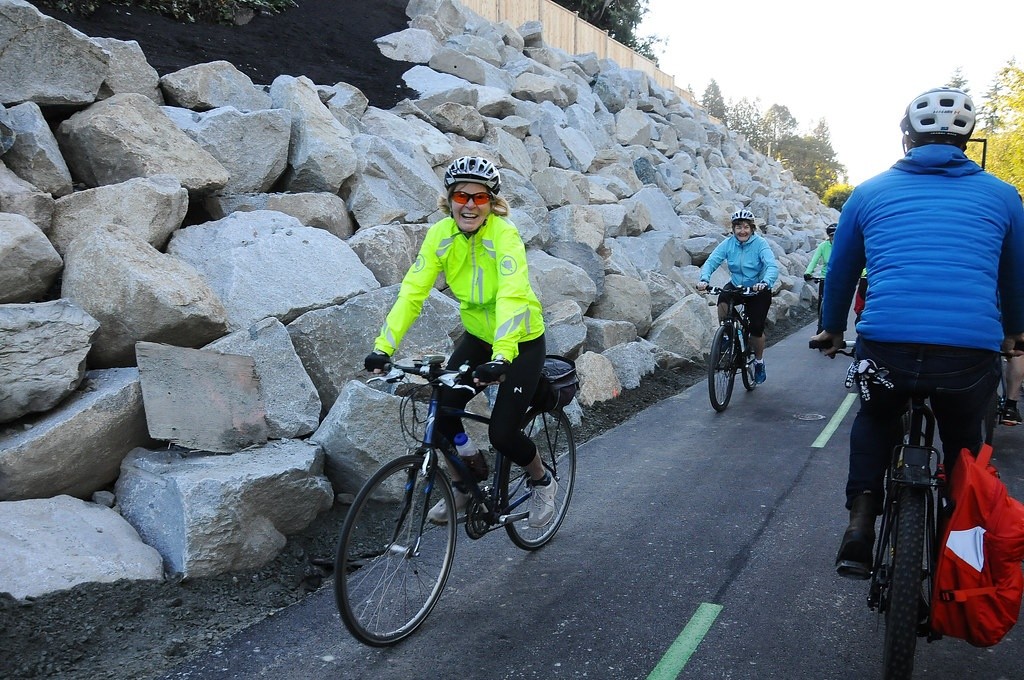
[450,191,491,206]
[829,234,834,237]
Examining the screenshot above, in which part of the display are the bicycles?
[694,281,770,412]
[809,339,1024,680]
[806,276,827,330]
[333,352,577,649]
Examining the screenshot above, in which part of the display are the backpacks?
[929,444,1024,649]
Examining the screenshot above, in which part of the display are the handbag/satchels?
[530,354,580,413]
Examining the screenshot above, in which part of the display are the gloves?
[803,274,811,281]
[697,282,710,293]
[753,282,770,291]
[845,358,894,401]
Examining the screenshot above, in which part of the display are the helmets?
[444,156,501,195]
[827,223,839,235]
[731,210,755,224]
[900,87,976,150]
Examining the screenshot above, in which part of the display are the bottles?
[454,434,489,484]
[738,330,745,352]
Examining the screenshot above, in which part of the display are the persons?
[803,222,838,336]
[363,157,559,528]
[853,267,868,326]
[697,210,779,383]
[813,84,1024,580]
[1001,340,1024,425]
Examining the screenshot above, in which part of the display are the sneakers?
[722,337,730,348]
[1002,407,1023,425]
[428,486,473,523]
[531,470,558,528]
[753,359,766,384]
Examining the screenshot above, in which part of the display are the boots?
[837,494,879,579]
